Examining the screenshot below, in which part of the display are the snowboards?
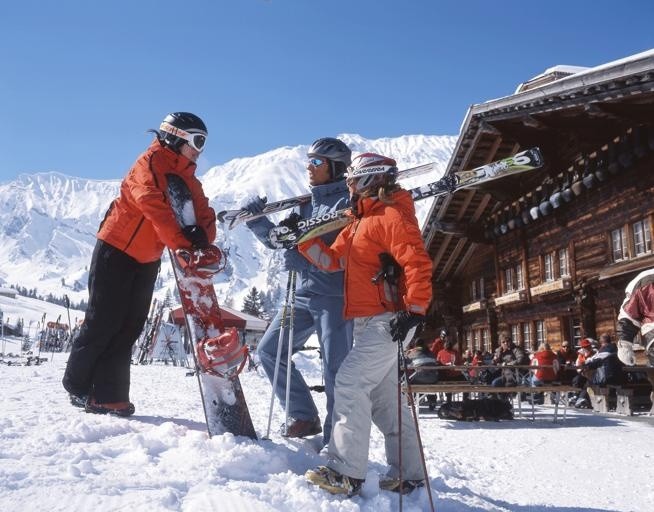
[163,176,255,436]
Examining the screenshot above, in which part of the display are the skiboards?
[44,327,67,353]
[1,352,47,366]
[267,146,544,251]
[132,298,190,367]
[218,161,437,231]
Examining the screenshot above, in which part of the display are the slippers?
[160,123,207,152]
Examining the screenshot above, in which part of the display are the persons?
[398,321,646,419]
[62,112,216,418]
[616,268,654,420]
[292,152,431,497]
[240,136,353,457]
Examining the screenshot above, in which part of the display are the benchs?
[401,362,653,422]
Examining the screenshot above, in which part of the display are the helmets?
[158,112,208,154]
[308,137,351,165]
[348,153,396,189]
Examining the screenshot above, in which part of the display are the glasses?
[311,159,335,166]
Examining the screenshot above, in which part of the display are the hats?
[580,340,590,347]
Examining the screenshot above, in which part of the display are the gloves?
[184,225,208,247]
[390,312,423,340]
[281,213,301,226]
[243,197,269,213]
[618,340,635,366]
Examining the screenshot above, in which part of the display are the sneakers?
[279,417,322,438]
[305,466,363,495]
[67,392,135,416]
[379,479,425,494]
[530,397,587,408]
